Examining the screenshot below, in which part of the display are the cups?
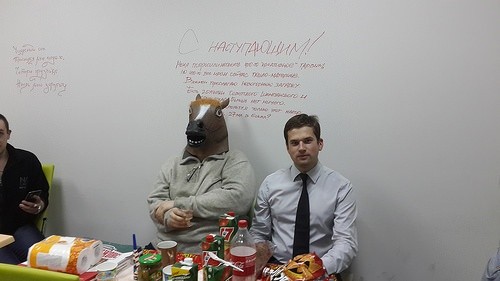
[95,261,119,281]
[162,265,173,281]
[157,240,178,266]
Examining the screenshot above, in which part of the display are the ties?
[292,173,310,273]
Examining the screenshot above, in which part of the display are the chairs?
[33,162,56,233]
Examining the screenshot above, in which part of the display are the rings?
[33,204,36,208]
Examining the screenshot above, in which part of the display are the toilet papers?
[27,234,104,275]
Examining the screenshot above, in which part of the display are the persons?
[0,114,50,265]
[147,94,257,252]
[248,114,359,281]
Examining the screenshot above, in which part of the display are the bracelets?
[35,206,41,214]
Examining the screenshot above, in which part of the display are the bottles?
[229,219,257,281]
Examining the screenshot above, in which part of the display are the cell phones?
[23,189,42,205]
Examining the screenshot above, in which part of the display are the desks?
[19,235,143,281]
